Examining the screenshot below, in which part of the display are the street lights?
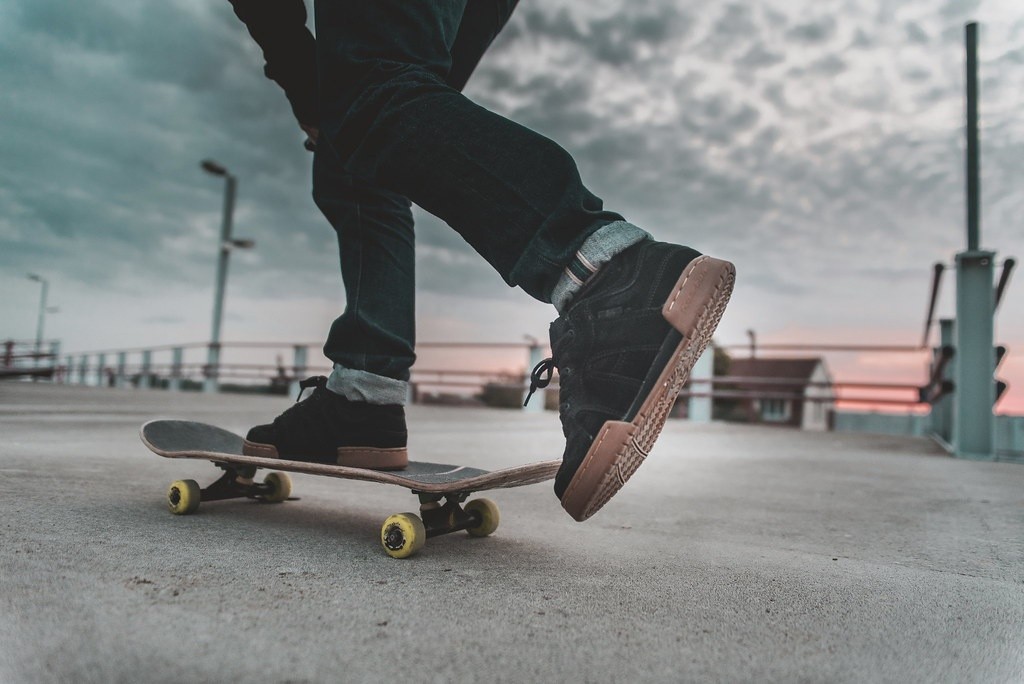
[199,153,240,398]
[24,270,48,382]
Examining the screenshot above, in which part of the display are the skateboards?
[139,418,564,559]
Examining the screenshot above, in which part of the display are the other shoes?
[242,376,408,468]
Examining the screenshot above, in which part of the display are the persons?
[230,0,738,522]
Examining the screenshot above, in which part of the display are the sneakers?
[524,237,736,522]
